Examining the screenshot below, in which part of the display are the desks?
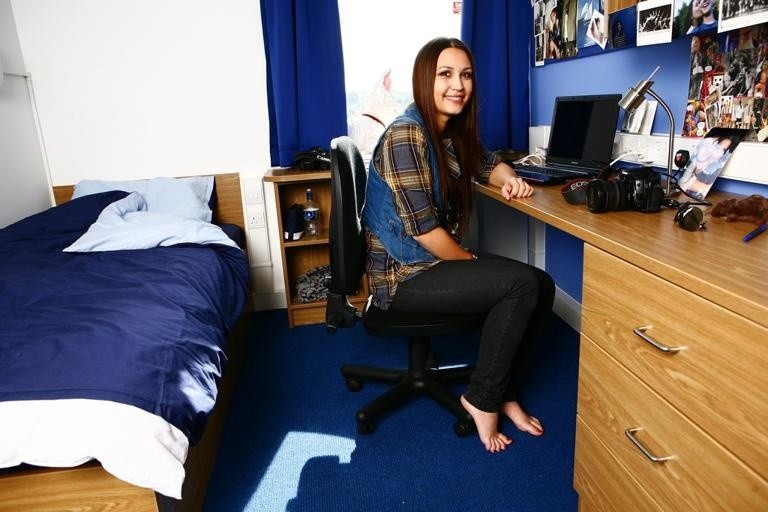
[461,164,768,511]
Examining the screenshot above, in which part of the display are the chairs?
[324,137,483,439]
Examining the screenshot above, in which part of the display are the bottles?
[302,188,322,241]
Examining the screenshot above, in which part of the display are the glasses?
[673,201,706,231]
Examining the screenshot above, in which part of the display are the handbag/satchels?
[296,147,331,170]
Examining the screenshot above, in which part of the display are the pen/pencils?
[743,224,768,241]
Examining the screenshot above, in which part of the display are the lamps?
[617,65,680,196]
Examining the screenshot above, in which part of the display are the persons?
[677,137,735,200]
[692,0,718,32]
[359,37,556,454]
[613,19,627,48]
[723,0,768,18]
[682,0,703,35]
[544,8,562,60]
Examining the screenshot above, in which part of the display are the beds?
[0,172,254,511]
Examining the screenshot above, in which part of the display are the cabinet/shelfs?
[261,166,369,329]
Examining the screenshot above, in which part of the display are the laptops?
[514,93,623,186]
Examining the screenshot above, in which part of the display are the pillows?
[69,176,216,223]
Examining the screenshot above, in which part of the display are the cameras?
[585,166,666,215]
